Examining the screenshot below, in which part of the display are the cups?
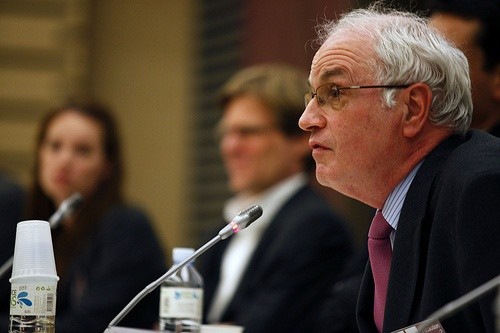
[9,220,60,282]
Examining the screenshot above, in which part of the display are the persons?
[194,64,362,333]
[0,102,167,333]
[426,0,500,139]
[299,0,500,333]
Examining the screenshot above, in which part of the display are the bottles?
[10,283,57,333]
[160,247,204,333]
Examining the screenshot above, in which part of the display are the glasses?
[304,82,410,109]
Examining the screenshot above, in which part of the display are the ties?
[368,209,393,333]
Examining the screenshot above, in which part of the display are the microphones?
[0,194,83,279]
[108,206,263,326]
